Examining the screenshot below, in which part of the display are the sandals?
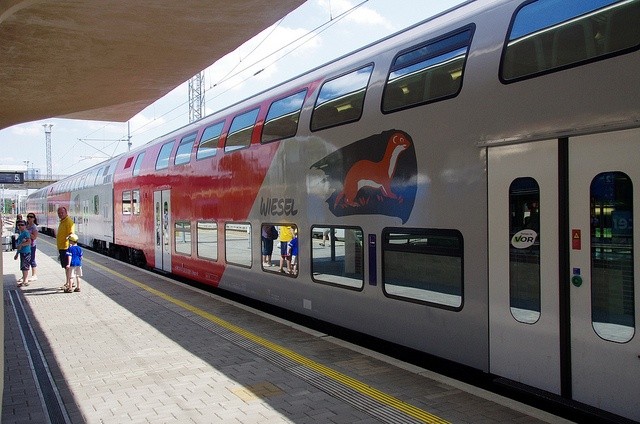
[60,283,69,290]
[64,282,75,287]
[64,288,72,293]
[17,282,29,287]
[74,288,80,292]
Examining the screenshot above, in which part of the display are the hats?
[66,234,78,242]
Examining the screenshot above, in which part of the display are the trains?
[20,0,640,424]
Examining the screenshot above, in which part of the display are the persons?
[262,225,277,267]
[16,214,23,233]
[287,228,298,276]
[278,225,295,273]
[19,213,38,281]
[64,234,83,292]
[56,206,75,290]
[14,220,31,287]
[319,230,331,247]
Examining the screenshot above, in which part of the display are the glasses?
[18,224,25,226]
[27,216,34,218]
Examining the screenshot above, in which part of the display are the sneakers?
[18,277,24,282]
[28,276,37,281]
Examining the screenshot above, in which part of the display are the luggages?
[12,235,17,250]
[2,236,12,252]
